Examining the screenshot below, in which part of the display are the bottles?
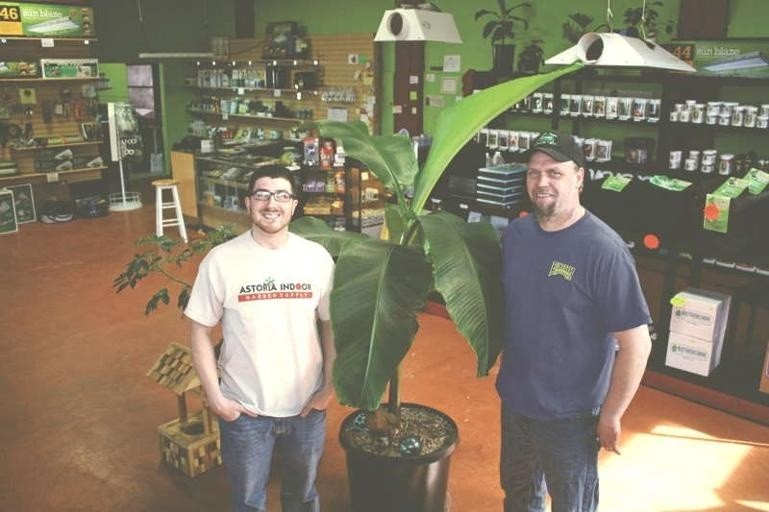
[485,152,492,167]
[493,152,504,166]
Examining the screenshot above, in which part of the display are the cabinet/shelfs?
[170,54,381,238]
[429,67,768,421]
[1,35,109,190]
[343,151,430,238]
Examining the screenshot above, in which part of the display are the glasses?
[248,189,296,203]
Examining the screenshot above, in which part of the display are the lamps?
[372,0,465,45]
[137,0,215,59]
[543,0,699,73]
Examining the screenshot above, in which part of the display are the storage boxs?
[663,335,725,376]
[668,287,736,342]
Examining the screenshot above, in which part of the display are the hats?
[520,130,586,169]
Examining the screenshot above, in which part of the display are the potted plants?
[287,62,586,512]
[516,39,546,73]
[474,0,532,72]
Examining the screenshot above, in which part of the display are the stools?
[152,177,191,244]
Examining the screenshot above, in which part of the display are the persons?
[495,129,654,512]
[183,165,337,511]
